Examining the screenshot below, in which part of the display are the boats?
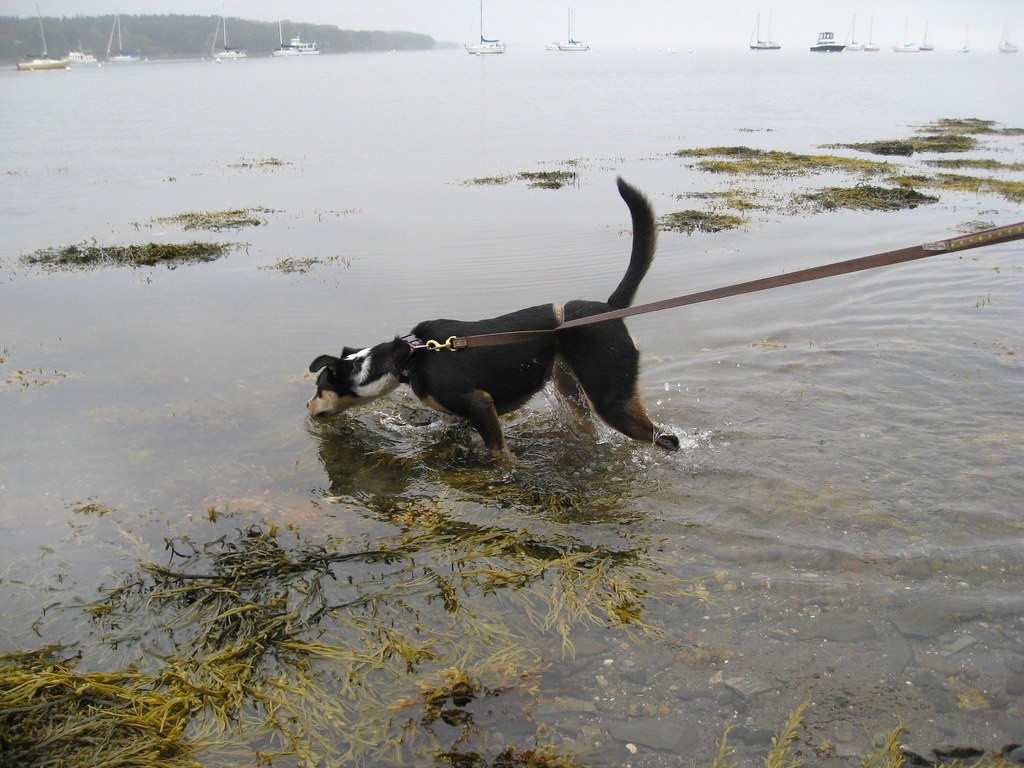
[810,32,847,53]
[546,45,553,50]
[272,37,321,55]
[61,40,98,65]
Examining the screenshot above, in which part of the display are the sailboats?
[748,7,781,49]
[465,0,505,54]
[860,18,881,50]
[16,3,71,70]
[208,5,248,58]
[917,20,935,50]
[558,5,591,52]
[104,11,140,62]
[843,13,864,51]
[891,17,920,53]
[999,17,1019,52]
[956,25,970,52]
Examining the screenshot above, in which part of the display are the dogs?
[305,174,681,458]
